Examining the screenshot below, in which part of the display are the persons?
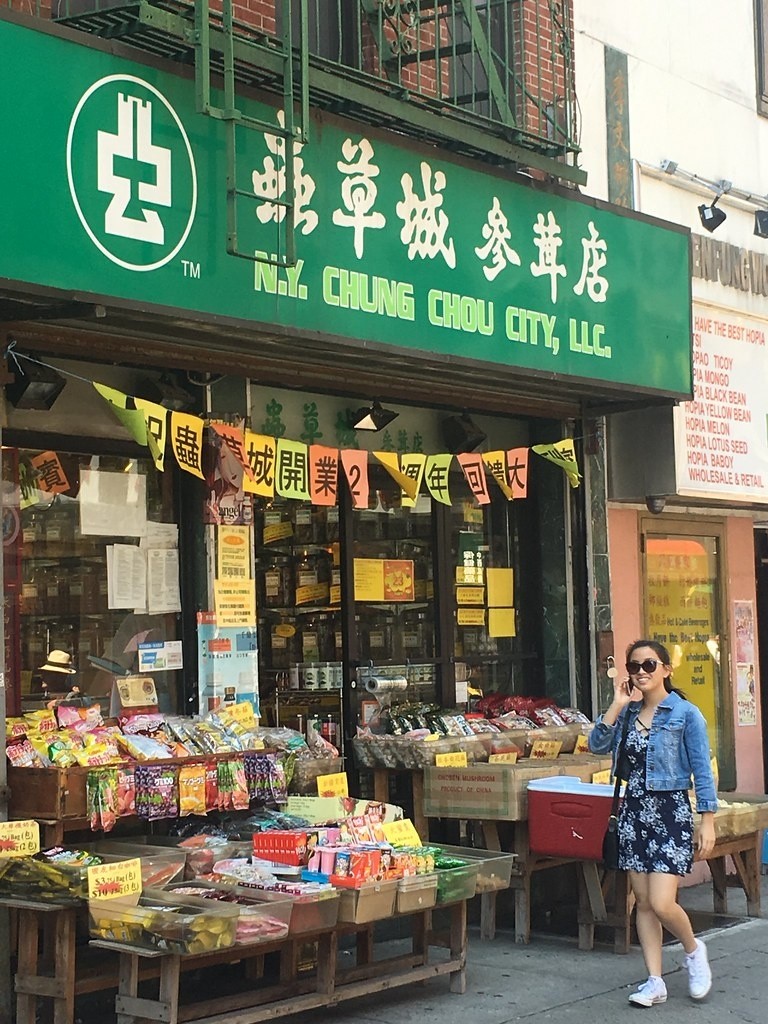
[38,650,76,701]
[87,609,179,715]
[589,640,717,1007]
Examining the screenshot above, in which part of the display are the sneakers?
[682,938,713,1000]
[628,975,668,1008]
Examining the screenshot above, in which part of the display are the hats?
[37,650,76,675]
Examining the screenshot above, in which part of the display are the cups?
[455,662,471,681]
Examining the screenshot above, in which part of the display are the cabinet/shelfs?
[0,501,186,700]
[352,736,761,954]
[0,733,472,1024]
[256,498,519,721]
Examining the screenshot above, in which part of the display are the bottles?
[291,713,336,748]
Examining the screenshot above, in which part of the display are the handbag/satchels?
[601,811,620,870]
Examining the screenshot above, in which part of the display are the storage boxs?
[1,834,519,955]
[357,723,767,860]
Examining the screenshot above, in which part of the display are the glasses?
[624,658,663,674]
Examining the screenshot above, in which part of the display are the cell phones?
[626,678,634,696]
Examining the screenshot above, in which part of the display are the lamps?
[661,160,726,234]
[718,179,768,239]
